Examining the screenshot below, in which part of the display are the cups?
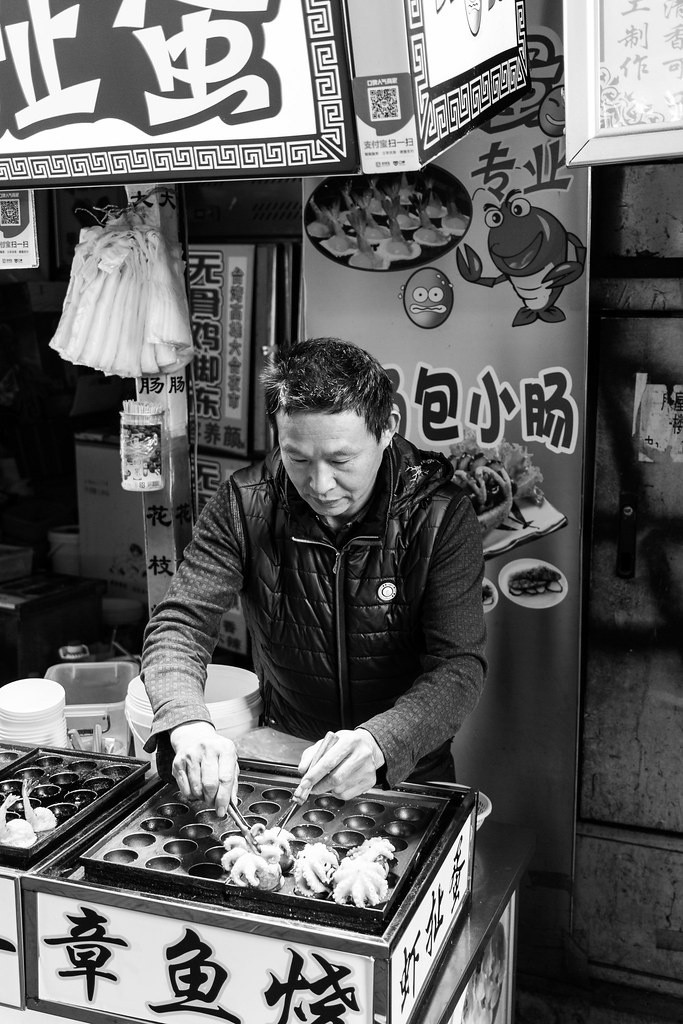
[116,411,168,494]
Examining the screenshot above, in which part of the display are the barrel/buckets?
[46,524,81,577]
[124,663,265,761]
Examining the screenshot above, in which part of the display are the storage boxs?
[0,544,33,583]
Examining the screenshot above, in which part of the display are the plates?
[482,577,498,613]
[0,677,71,750]
[498,557,568,609]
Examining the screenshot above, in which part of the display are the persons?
[140,339,489,818]
